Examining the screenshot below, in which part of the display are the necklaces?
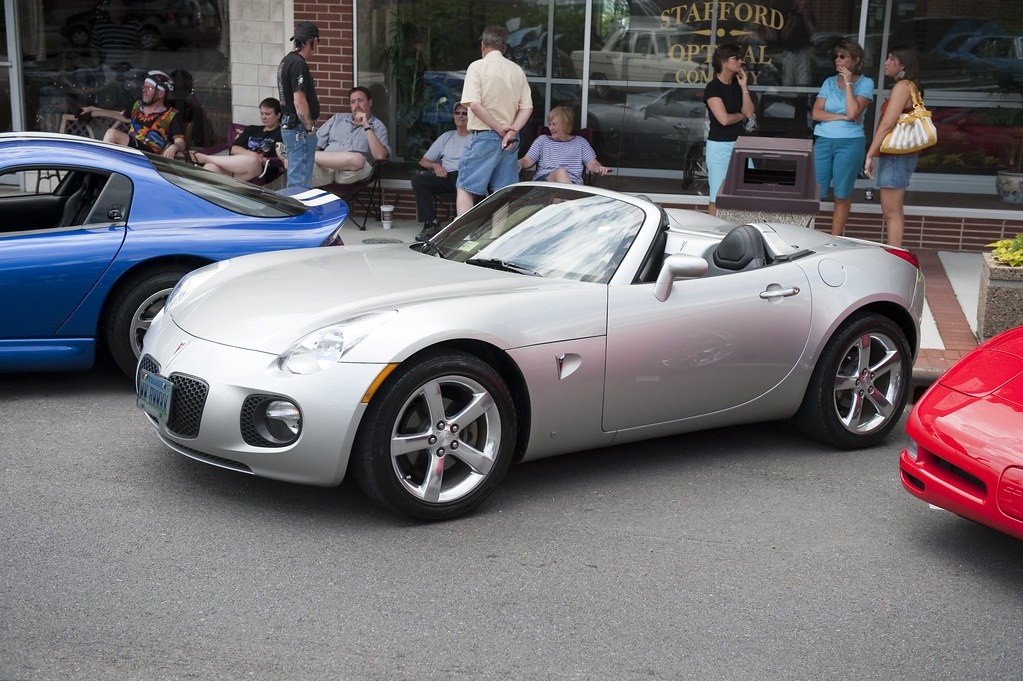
[717,75,732,84]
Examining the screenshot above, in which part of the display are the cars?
[811,17,1023,94]
[355,22,725,171]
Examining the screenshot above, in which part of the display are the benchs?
[60,110,197,160]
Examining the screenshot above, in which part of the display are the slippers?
[184,148,205,167]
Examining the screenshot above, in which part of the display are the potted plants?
[971,26,1023,203]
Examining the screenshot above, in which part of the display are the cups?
[381,205,394,229]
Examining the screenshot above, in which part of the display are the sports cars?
[135,179,925,520]
[896,321,1023,541]
[0,131,348,384]
[925,106,1023,165]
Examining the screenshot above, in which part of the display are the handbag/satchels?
[879,80,938,153]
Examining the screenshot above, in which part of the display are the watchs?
[308,125,316,133]
[364,125,374,132]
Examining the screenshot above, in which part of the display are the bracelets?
[846,82,853,85]
[743,91,748,94]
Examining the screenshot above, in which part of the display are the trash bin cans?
[715,135,820,229]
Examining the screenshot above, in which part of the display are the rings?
[845,72,847,74]
[864,171,867,173]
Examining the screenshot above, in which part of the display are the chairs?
[418,165,484,228]
[699,225,766,275]
[57,174,103,227]
[320,157,390,230]
[184,123,287,187]
[538,127,614,185]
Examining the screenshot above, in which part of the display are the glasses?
[726,55,743,61]
[310,37,320,43]
[141,85,159,92]
[834,54,853,60]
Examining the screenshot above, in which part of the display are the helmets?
[144,70,175,94]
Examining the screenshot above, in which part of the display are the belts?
[473,129,489,134]
[454,111,467,116]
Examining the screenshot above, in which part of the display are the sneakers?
[415,220,442,241]
[274,141,289,162]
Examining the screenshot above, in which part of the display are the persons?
[455,24,533,241]
[89,0,189,107]
[411,101,473,242]
[812,38,874,235]
[276,22,320,189]
[517,106,612,204]
[187,97,287,182]
[79,70,186,159]
[703,44,755,218]
[864,44,924,248]
[262,87,392,192]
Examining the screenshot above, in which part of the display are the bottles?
[865,189,874,200]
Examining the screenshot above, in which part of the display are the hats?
[290,21,319,41]
[453,102,461,110]
